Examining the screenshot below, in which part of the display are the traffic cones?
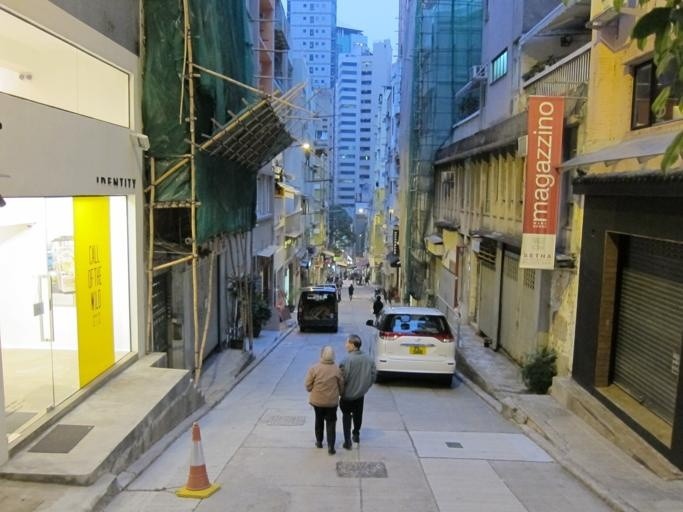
[175,422,223,501]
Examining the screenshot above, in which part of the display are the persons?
[335,279,343,303]
[348,283,354,306]
[338,332,378,450]
[357,319,379,376]
[305,344,345,455]
[372,295,383,324]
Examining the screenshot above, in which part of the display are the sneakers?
[328,447,335,454]
[352,437,360,442]
[315,442,323,448]
[343,443,352,449]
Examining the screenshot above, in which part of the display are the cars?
[318,284,341,301]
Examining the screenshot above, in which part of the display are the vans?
[296,286,339,334]
[366,306,457,388]
